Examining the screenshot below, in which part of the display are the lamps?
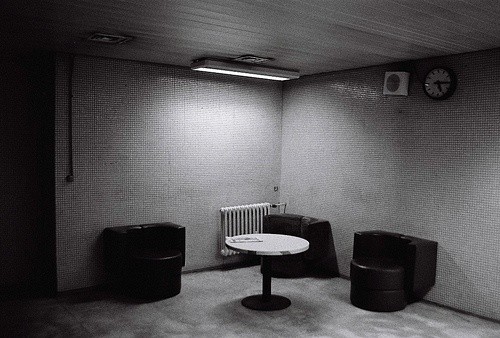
[190,58,300,81]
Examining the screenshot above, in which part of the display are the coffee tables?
[225,233,309,310]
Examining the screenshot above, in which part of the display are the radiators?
[220,202,272,257]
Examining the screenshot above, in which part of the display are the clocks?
[422,65,457,100]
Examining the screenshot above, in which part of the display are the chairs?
[260,215,331,278]
[349,229,439,313]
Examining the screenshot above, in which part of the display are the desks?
[102,222,185,300]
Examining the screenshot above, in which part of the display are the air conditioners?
[383,70,411,96]
